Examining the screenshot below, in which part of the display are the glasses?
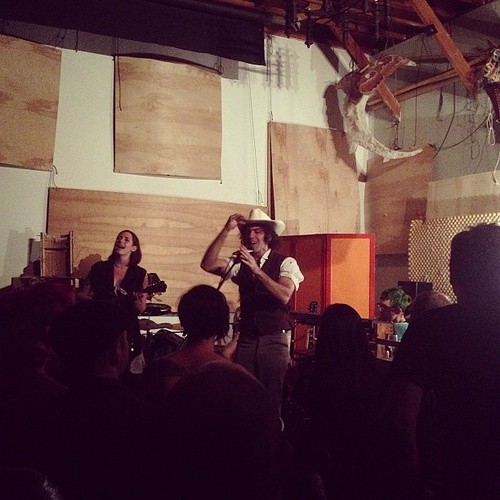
[404,305,414,322]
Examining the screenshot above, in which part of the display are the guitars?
[79,281,167,304]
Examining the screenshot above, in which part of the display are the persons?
[200,208,304,431]
[0,223,500,500]
[75,230,148,357]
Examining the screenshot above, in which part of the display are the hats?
[237,208,285,237]
[65,300,129,361]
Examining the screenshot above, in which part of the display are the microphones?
[233,246,255,256]
